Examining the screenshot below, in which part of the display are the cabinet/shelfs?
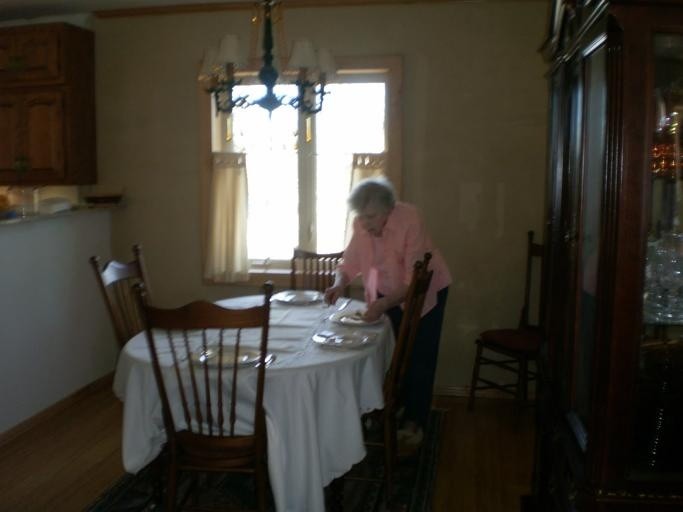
[0,21,99,185]
[537,0,683,512]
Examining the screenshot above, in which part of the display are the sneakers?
[397,425,423,446]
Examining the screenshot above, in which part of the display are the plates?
[310,329,377,348]
[191,348,261,366]
[330,310,385,327]
[269,290,319,304]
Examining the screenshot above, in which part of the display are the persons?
[324,180,453,444]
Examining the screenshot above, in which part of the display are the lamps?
[208,0,326,118]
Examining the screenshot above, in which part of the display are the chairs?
[90,244,147,348]
[324,251,433,511]
[131,279,276,512]
[291,250,351,298]
[469,229,546,424]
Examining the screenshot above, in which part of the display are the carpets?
[74,417,442,512]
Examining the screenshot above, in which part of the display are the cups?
[6,186,39,219]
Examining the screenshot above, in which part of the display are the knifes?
[338,298,353,311]
